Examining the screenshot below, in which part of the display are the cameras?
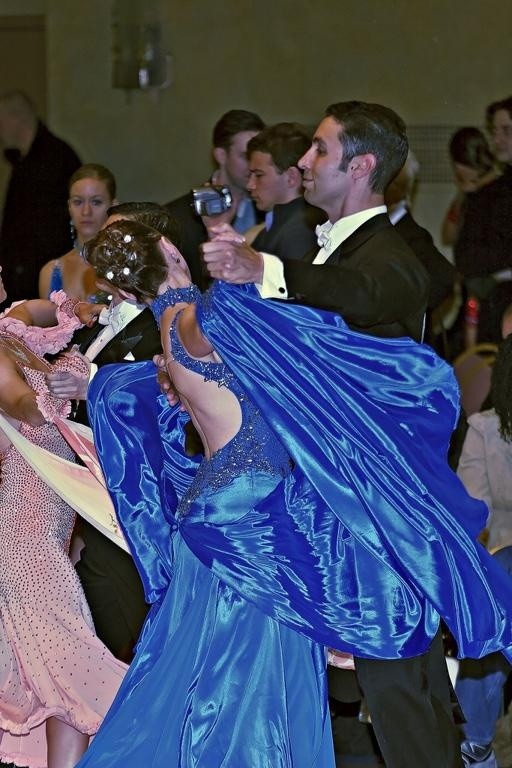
[191,182,235,218]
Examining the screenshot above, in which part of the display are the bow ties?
[315,225,331,251]
